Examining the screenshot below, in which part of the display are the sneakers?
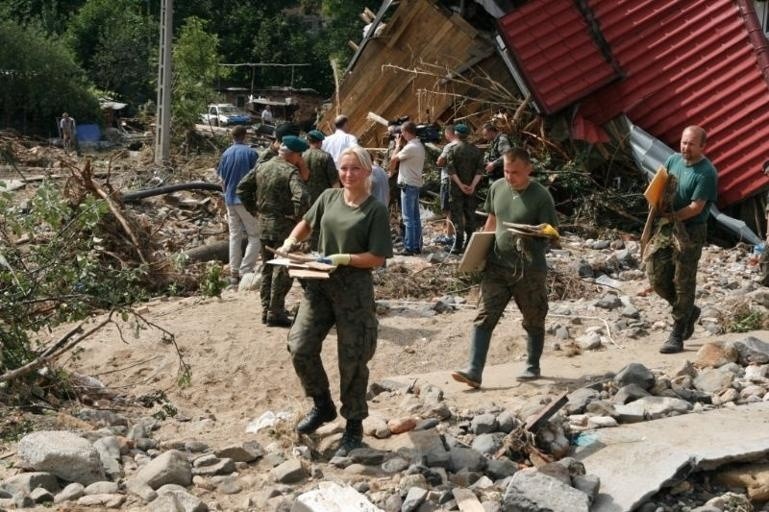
[659,333,686,354]
[393,247,424,257]
[265,311,294,328]
[448,234,478,254]
[230,270,242,284]
[261,309,289,324]
[685,304,702,341]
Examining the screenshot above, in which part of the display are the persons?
[452,149,561,390]
[640,125,718,353]
[60,112,82,158]
[273,147,394,456]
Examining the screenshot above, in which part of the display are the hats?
[274,121,301,140]
[308,129,326,142]
[454,124,471,135]
[281,135,310,153]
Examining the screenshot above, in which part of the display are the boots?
[449,323,492,389]
[297,399,339,434]
[515,329,545,382]
[333,418,366,459]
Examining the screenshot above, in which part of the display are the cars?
[199,101,252,128]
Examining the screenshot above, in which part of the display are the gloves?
[323,252,351,276]
[272,236,302,260]
